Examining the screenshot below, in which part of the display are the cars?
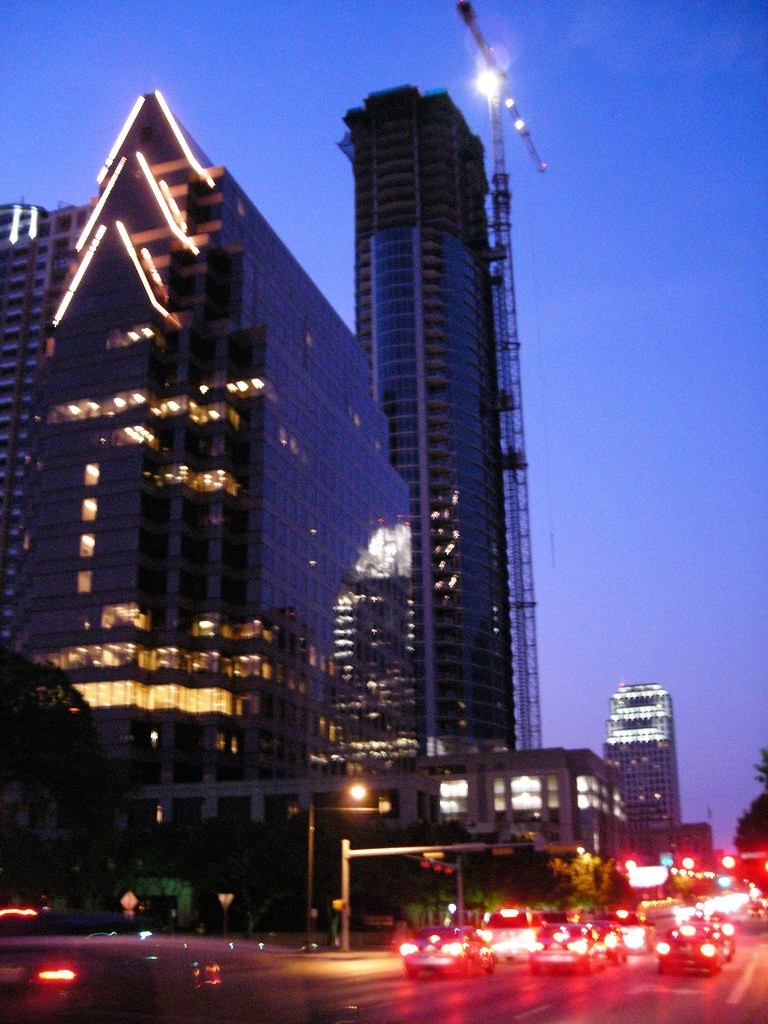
[595,921,630,966]
[0,906,222,1024]
[619,915,658,952]
[658,925,724,974]
[402,925,497,978]
[482,910,544,960]
[527,922,610,976]
[700,922,737,962]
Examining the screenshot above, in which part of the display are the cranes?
[451,0,551,752]
